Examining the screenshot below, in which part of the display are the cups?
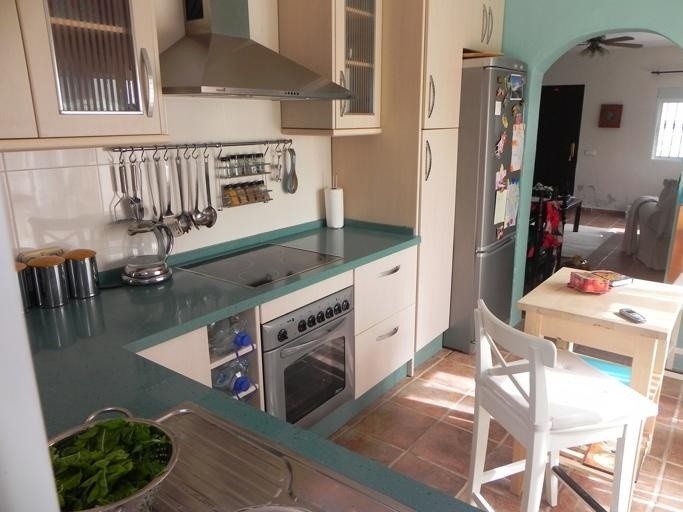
[12,250,100,314]
[24,295,105,353]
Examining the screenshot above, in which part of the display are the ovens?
[261,285,356,441]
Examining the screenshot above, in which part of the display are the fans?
[577,34,646,60]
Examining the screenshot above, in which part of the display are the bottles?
[221,153,271,207]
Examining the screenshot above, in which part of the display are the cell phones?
[620,308,646,322]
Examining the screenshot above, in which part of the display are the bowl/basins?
[46,406,181,512]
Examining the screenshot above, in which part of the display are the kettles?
[120,220,174,286]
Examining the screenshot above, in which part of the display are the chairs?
[471,298,658,511]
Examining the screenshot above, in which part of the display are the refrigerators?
[442,56,529,354]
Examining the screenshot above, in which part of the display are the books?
[595,269,634,287]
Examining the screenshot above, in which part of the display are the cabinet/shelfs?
[136,307,267,414]
[278,0,506,401]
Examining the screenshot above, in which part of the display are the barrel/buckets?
[208,316,254,361]
[210,361,259,402]
